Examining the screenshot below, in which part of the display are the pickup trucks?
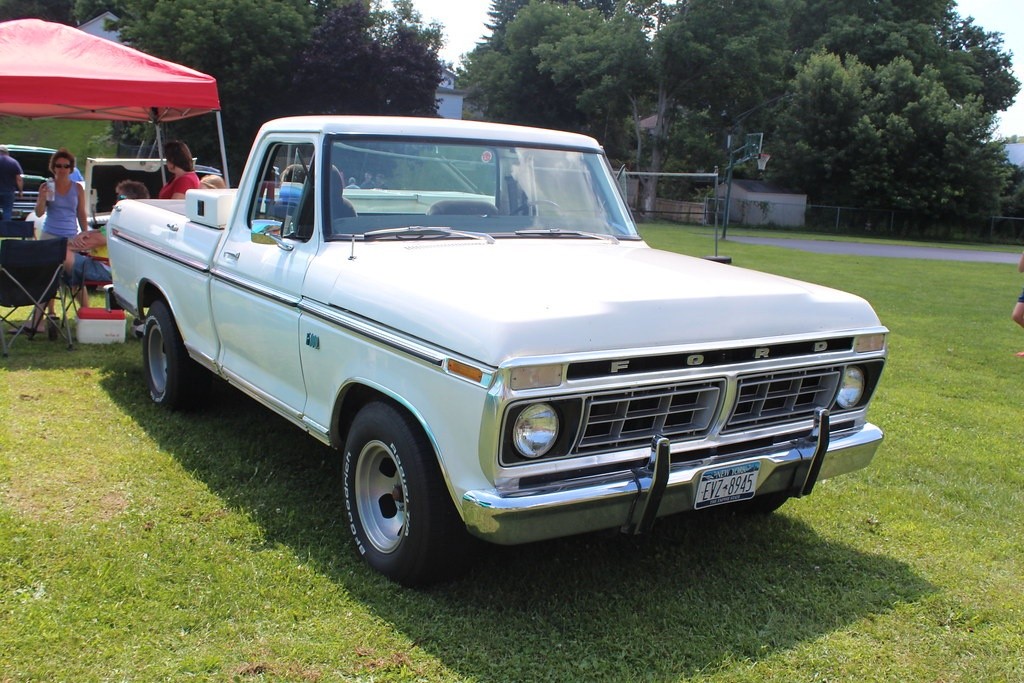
[104,111,888,583]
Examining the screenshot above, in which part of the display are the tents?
[0,18,221,126]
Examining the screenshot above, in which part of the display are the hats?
[0,145,8,152]
[200,175,225,189]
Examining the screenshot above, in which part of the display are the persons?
[158,141,201,200]
[22,179,150,333]
[200,175,227,189]
[344,171,387,189]
[1009,251,1024,357]
[34,146,91,321]
[0,145,23,223]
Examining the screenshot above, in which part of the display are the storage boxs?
[77,308,127,345]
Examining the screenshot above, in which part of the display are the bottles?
[45,177,55,201]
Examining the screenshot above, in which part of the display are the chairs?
[0,221,35,239]
[427,199,499,217]
[61,252,111,328]
[297,165,357,235]
[0,235,73,357]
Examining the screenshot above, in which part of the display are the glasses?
[117,194,127,200]
[55,163,72,168]
